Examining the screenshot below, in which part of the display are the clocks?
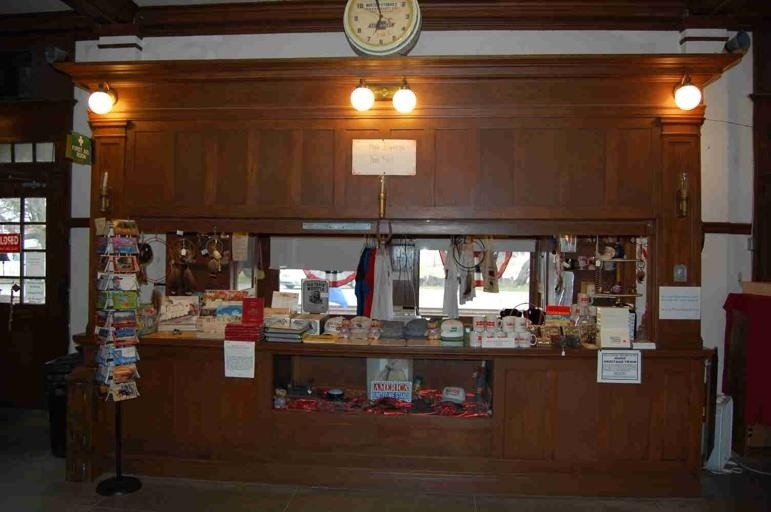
[342,0,423,57]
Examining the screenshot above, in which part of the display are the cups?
[578,254,590,265]
[468,313,539,348]
[571,293,597,317]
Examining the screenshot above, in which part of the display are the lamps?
[85,81,117,115]
[348,77,418,114]
[673,73,704,110]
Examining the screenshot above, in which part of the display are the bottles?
[574,314,601,352]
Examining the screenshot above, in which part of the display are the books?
[141,289,338,345]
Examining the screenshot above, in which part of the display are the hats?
[439,387,464,404]
[440,320,464,340]
[322,316,428,339]
